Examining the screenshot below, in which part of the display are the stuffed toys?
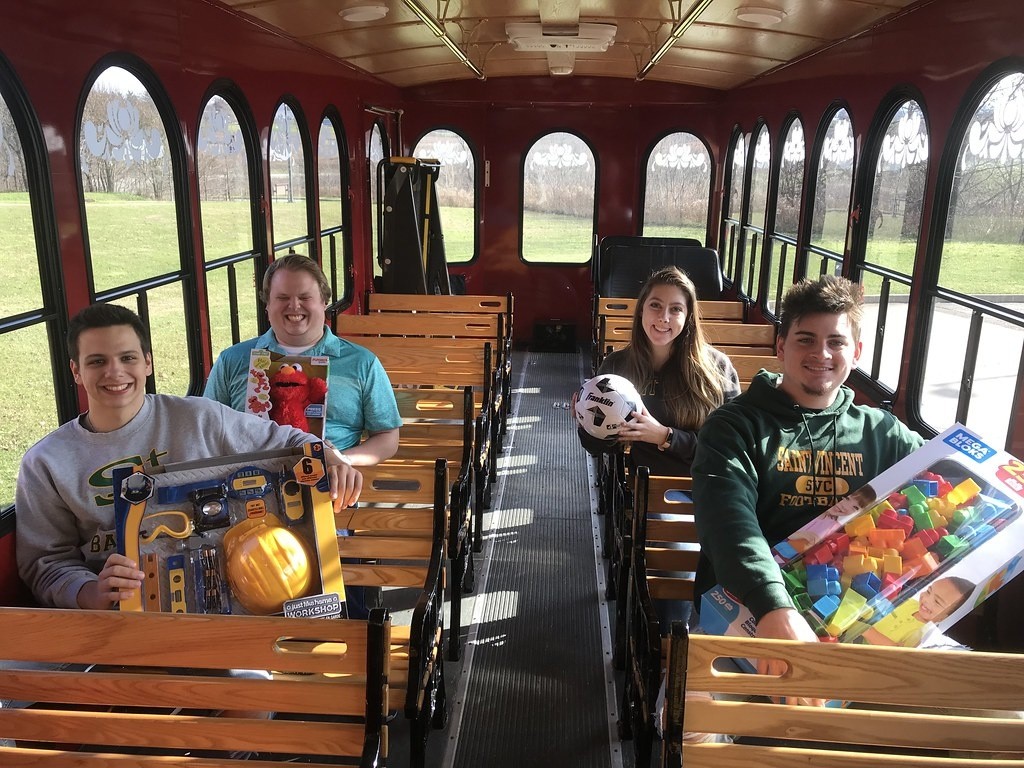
[269,360,327,432]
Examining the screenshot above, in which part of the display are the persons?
[691,275,978,761]
[569,265,741,624]
[198,254,403,722]
[861,575,976,647]
[13,302,363,758]
[786,482,878,546]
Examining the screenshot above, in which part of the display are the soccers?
[574,374,648,440]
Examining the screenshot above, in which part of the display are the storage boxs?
[699,421,1024,709]
[112,441,346,615]
[246,349,329,441]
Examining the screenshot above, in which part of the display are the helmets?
[223,512,321,618]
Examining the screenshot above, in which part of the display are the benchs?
[665,621,1024,768]
[331,457,450,767]
[601,296,756,601]
[391,382,479,664]
[340,336,490,557]
[331,306,505,491]
[627,466,978,722]
[614,346,796,668]
[364,288,515,415]
[597,241,723,319]
[0,604,396,768]
[596,321,777,518]
